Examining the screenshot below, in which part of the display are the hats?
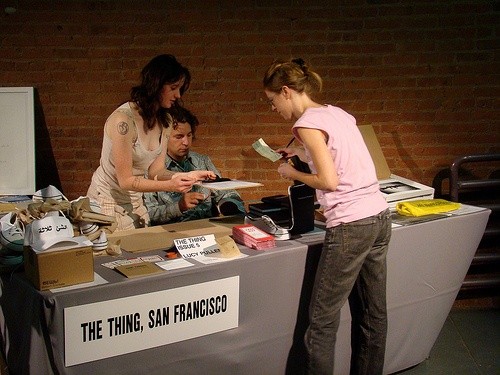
[23,216,79,251]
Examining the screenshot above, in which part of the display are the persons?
[87,53,216,233]
[142,102,248,226]
[262,56,391,375]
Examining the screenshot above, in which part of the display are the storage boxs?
[23,230,94,291]
[358,125,435,207]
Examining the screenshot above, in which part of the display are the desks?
[0,200,491,375]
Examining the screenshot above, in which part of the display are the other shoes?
[32,185,108,251]
[1,255,23,265]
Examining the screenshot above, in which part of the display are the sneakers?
[0,211,24,251]
